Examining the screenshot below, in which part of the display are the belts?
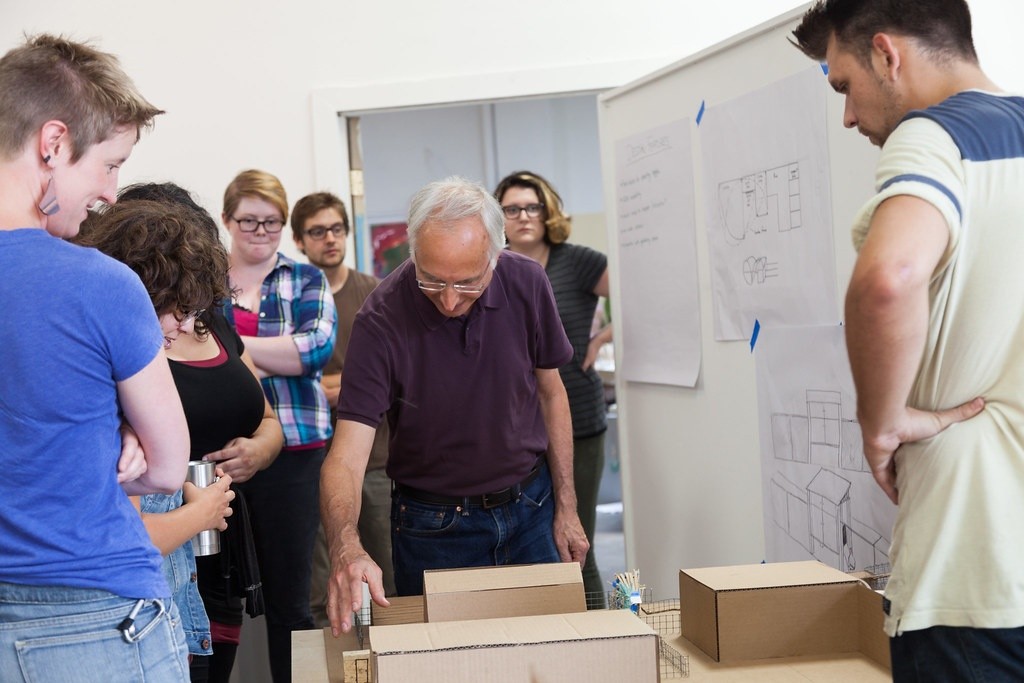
[394,454,546,508]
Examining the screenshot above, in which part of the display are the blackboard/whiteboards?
[596,0,1024,603]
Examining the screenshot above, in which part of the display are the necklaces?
[535,245,547,264]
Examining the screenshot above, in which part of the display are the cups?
[188,461,221,556]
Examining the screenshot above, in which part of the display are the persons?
[114,183,286,683]
[489,171,613,614]
[0,33,197,683]
[786,0,1024,683]
[218,168,338,683]
[288,193,400,632]
[315,174,592,640]
[68,196,237,665]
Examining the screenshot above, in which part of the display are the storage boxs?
[679,560,892,670]
[368,609,661,683]
[424,562,587,623]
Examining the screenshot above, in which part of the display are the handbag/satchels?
[232,485,257,589]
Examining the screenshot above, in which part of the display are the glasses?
[179,309,205,326]
[502,203,544,219]
[232,214,286,233]
[301,223,345,240]
[415,250,494,293]
